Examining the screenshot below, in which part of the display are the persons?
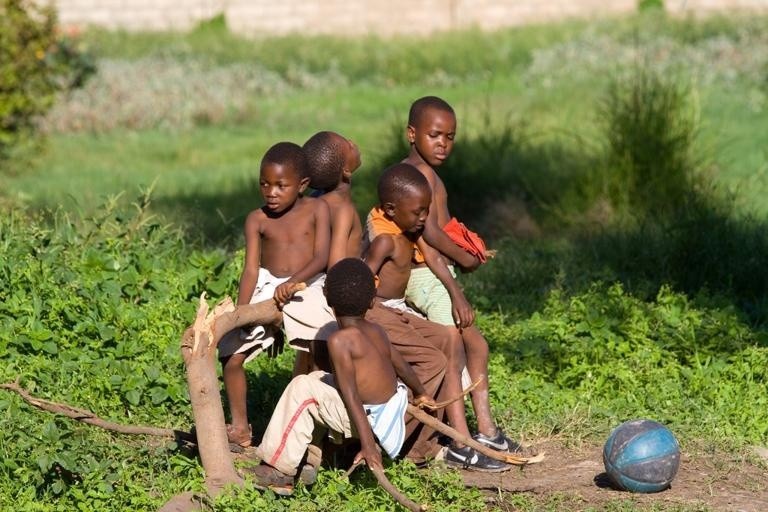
[217,97,530,472]
[238,257,435,498]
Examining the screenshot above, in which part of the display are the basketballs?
[602,419,680,493]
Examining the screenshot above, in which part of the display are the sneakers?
[297,463,317,486]
[445,441,507,473]
[237,465,295,496]
[474,429,525,455]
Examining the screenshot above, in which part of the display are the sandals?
[227,424,252,453]
[408,441,446,466]
